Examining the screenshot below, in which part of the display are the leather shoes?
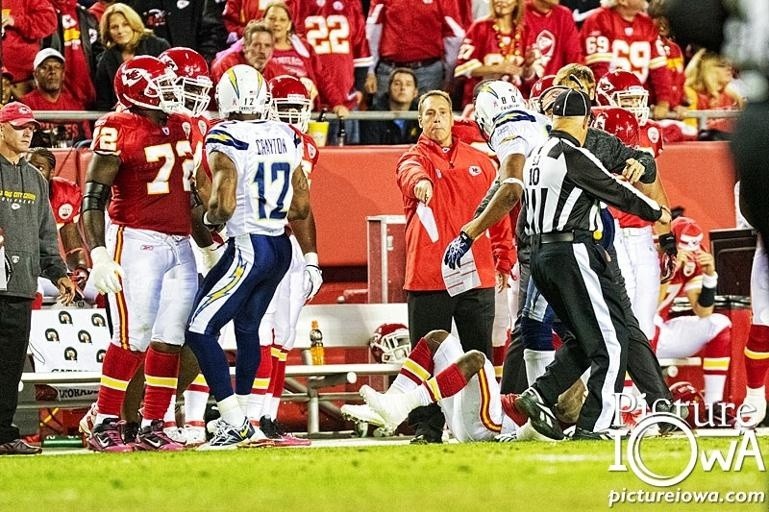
[0,439,42,455]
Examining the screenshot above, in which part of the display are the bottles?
[309,320,326,366]
[317,108,327,122]
[336,115,348,146]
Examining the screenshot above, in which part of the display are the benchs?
[204,293,707,441]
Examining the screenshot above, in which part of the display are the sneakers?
[135,420,186,451]
[733,384,766,429]
[658,403,688,435]
[163,428,205,448]
[704,400,737,426]
[78,404,99,448]
[273,419,311,446]
[249,415,300,447]
[495,420,562,441]
[572,426,628,441]
[196,417,255,450]
[514,387,564,440]
[359,384,405,430]
[87,418,133,452]
[340,403,385,427]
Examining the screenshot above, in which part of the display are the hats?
[670,216,703,251]
[33,48,65,70]
[0,101,41,128]
[553,88,591,115]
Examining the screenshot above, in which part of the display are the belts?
[380,58,441,68]
[531,234,573,244]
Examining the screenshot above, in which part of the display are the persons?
[0,0,767,456]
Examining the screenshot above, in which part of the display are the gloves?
[90,247,124,296]
[444,231,474,270]
[302,253,323,300]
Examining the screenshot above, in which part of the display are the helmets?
[215,64,267,118]
[366,323,411,364]
[527,75,558,113]
[268,75,311,133]
[595,70,649,126]
[475,80,527,135]
[158,47,213,118]
[114,55,184,114]
[591,109,639,149]
